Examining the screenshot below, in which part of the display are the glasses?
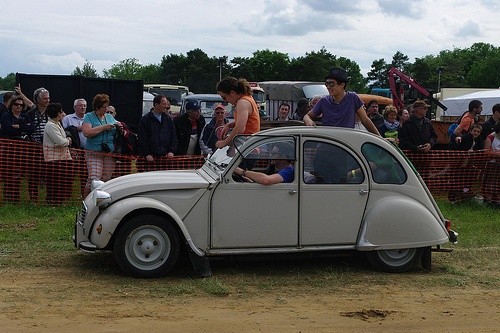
[14,102,24,107]
[324,81,335,87]
[106,111,114,114]
[272,151,280,156]
[35,89,47,99]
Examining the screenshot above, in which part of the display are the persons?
[215,76,260,182]
[0,83,233,207]
[257,95,500,204]
[234,141,295,185]
[303,69,401,184]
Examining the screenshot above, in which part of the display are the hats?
[214,104,225,110]
[186,100,202,111]
[325,66,348,82]
[413,100,431,108]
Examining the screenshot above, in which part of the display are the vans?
[141,80,500,150]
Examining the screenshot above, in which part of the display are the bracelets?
[351,170,356,177]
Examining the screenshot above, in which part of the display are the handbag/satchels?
[448,111,473,151]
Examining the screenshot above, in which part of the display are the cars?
[73,124,459,280]
